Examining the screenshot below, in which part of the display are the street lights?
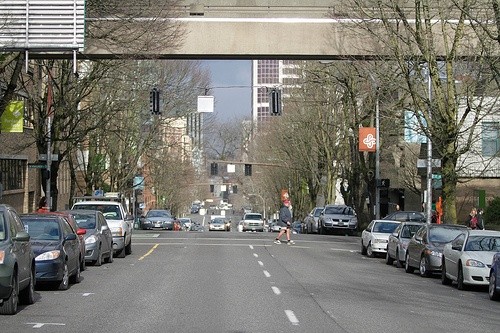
[318,60,382,219]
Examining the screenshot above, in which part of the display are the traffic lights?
[380,179,390,187]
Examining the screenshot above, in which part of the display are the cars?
[55,210,114,266]
[360,210,436,257]
[191,199,206,214]
[317,204,359,235]
[173,217,204,232]
[385,222,426,268]
[488,253,500,300]
[237,206,324,234]
[405,223,472,277]
[141,210,176,230]
[207,218,232,232]
[440,229,500,291]
[0,204,37,316]
[16,208,87,290]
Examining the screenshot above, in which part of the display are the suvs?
[70,200,136,258]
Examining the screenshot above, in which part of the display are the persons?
[273,201,295,245]
[475,208,485,230]
[38,197,47,209]
[465,208,478,230]
